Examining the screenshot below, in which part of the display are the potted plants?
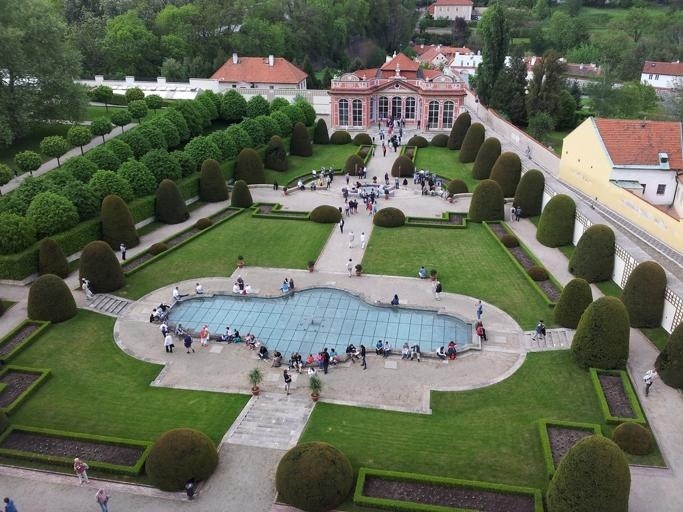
[309,376,322,402]
[249,368,262,395]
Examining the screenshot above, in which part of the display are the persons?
[272,114,454,250]
[94,487,111,512]
[419,266,427,278]
[347,259,354,278]
[515,206,522,222]
[185,477,196,500]
[119,243,126,261]
[72,456,90,486]
[150,275,489,377]
[4,496,16,511]
[283,368,292,396]
[643,365,656,396]
[81,278,93,300]
[531,318,542,341]
[510,204,516,221]
